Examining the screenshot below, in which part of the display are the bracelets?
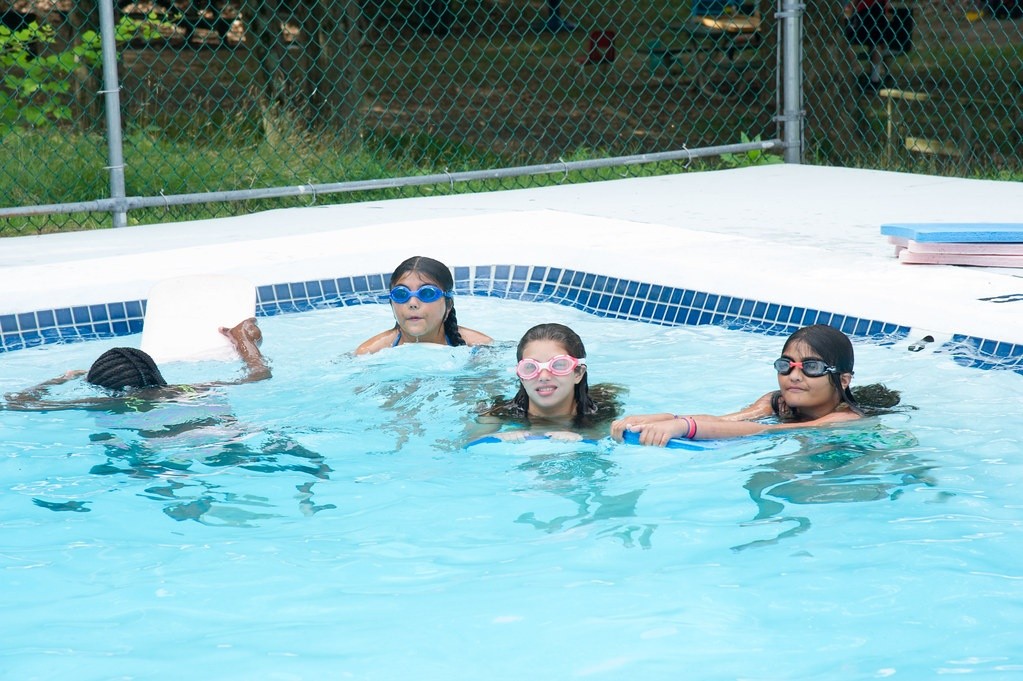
[665,412,698,440]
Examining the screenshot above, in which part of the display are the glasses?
[389,285,451,304]
[774,357,854,379]
[516,355,585,379]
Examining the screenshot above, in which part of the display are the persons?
[610,323,866,449]
[3,317,273,412]
[465,323,621,444]
[352,255,495,358]
